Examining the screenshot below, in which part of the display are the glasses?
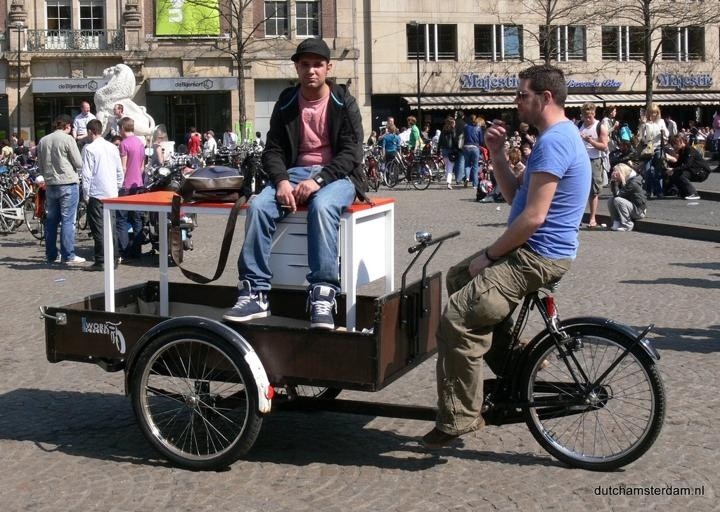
[516,90,543,100]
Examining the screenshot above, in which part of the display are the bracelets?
[485,250,500,263]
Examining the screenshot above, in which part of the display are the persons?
[222,39,370,328]
[420,65,593,450]
[367,101,720,232]
[254,131,265,152]
[1,100,238,271]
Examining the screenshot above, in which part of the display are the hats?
[291,39,331,61]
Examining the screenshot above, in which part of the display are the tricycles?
[36,230,667,470]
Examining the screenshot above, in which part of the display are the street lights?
[410,19,423,133]
[13,21,24,146]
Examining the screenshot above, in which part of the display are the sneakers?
[423,414,485,449]
[62,256,86,264]
[222,279,271,321]
[306,285,338,329]
[685,193,700,200]
[82,262,104,271]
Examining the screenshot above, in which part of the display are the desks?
[101,191,398,335]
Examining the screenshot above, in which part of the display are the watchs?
[313,174,326,187]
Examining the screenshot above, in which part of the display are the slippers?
[588,223,597,227]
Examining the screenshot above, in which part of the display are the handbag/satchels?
[656,158,670,176]
[182,166,252,200]
[448,152,458,162]
